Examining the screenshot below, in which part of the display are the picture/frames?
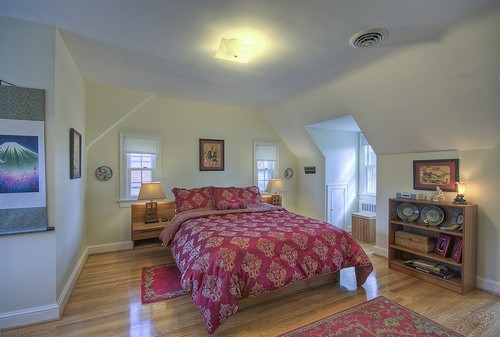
[413,159,459,192]
[199,138,225,171]
[70,128,82,179]
[435,233,463,263]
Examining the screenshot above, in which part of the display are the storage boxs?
[395,230,435,253]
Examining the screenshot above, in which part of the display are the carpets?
[275,295,465,337]
[141,263,189,304]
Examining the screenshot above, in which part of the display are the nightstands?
[131,201,175,241]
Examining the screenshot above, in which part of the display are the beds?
[159,203,374,334]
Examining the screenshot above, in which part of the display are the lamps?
[452,182,468,204]
[137,182,167,224]
[214,36,258,63]
[264,179,289,207]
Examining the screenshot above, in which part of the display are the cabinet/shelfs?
[388,198,478,295]
[352,211,376,243]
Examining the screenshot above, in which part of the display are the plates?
[452,207,464,230]
[397,203,419,221]
[421,205,444,225]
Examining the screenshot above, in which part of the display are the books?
[404,258,459,280]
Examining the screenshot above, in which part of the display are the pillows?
[172,186,264,214]
